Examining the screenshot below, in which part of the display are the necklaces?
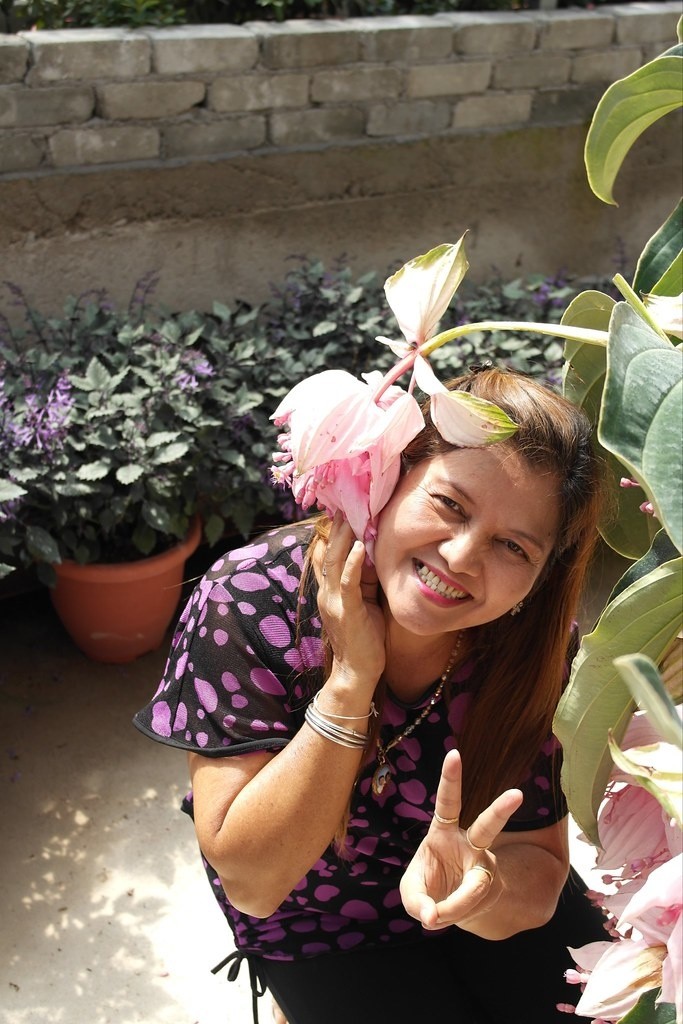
[371,628,465,795]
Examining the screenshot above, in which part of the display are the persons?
[131,366,623,1023]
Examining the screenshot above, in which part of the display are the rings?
[472,866,494,883]
[467,827,492,850]
[434,809,460,824]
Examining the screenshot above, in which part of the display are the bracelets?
[304,688,380,749]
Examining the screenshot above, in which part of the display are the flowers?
[0,270,218,589]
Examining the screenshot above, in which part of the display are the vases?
[33,511,203,665]
